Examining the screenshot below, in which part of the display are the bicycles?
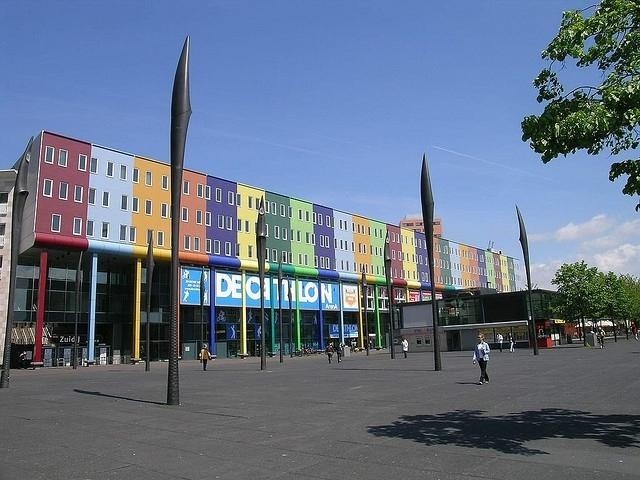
[597,335,605,349]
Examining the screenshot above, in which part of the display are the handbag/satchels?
[483,354,489,361]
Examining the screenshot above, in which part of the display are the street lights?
[287,281,292,358]
[199,266,206,360]
[254,194,268,372]
[514,204,540,356]
[277,261,285,362]
[362,270,371,357]
[382,229,396,359]
[70,250,84,371]
[1,135,36,390]
[144,233,155,372]
[417,151,443,370]
[163,29,193,404]
[487,282,491,288]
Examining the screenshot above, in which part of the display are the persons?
[595,326,606,344]
[495,332,504,352]
[473,333,491,385]
[336,341,343,364]
[508,332,516,353]
[401,338,409,360]
[325,342,335,365]
[198,344,212,371]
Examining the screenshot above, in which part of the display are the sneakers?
[485,381,489,384]
[479,381,483,385]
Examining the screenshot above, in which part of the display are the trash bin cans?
[537,335,552,349]
[585,332,598,346]
[342,346,351,357]
